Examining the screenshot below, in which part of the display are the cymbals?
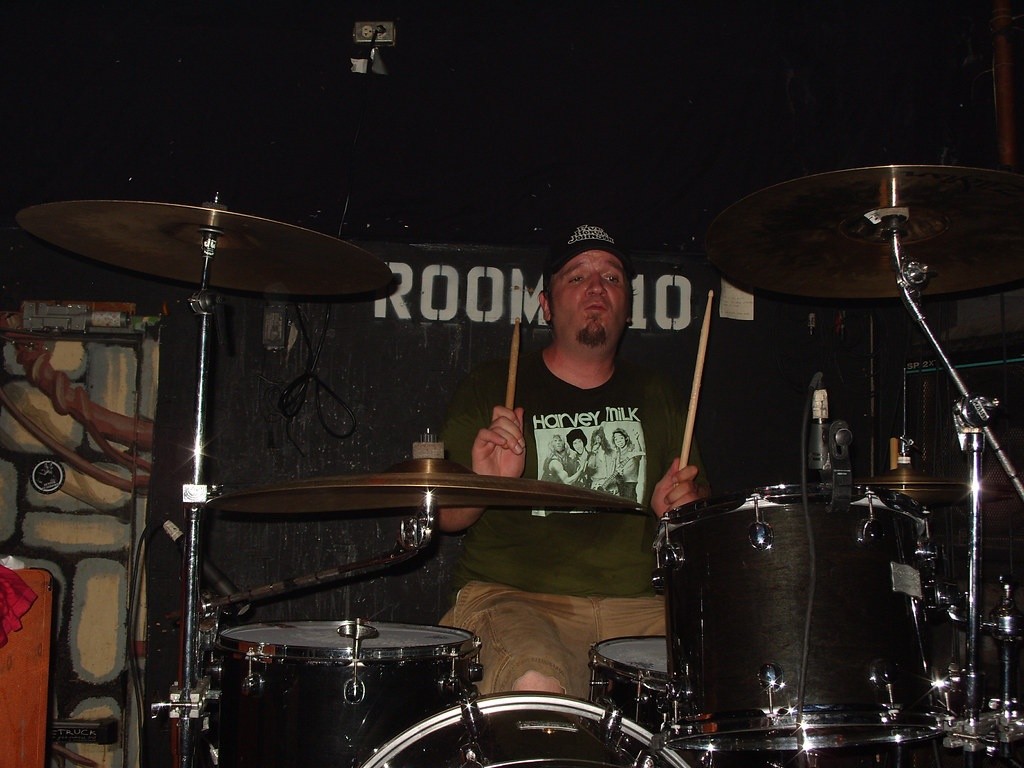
[199,458,652,515]
[852,467,1017,505]
[703,164,1024,301]
[14,198,392,297]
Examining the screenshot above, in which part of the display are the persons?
[438,217,712,737]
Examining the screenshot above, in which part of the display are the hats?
[544,222,632,283]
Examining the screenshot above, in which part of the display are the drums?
[352,689,694,768]
[583,632,671,738]
[207,618,483,768]
[653,482,951,753]
